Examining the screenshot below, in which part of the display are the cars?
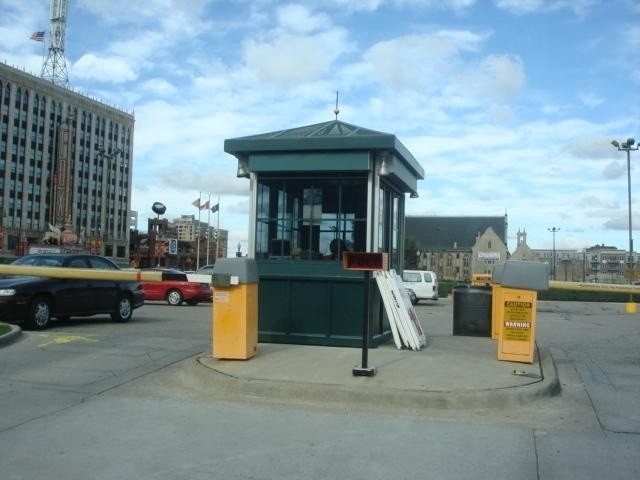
[141,268,211,306]
[0,253,145,331]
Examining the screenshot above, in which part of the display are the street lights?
[547,227,560,280]
[611,138,640,285]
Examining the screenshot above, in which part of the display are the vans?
[403,270,439,305]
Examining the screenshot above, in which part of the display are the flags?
[31,31,45,41]
[211,203,218,213]
[200,201,209,209]
[192,199,199,209]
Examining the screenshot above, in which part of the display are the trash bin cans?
[452,284,492,337]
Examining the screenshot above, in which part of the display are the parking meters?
[343,251,391,369]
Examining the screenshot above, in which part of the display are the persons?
[129,255,136,267]
[325,238,347,259]
